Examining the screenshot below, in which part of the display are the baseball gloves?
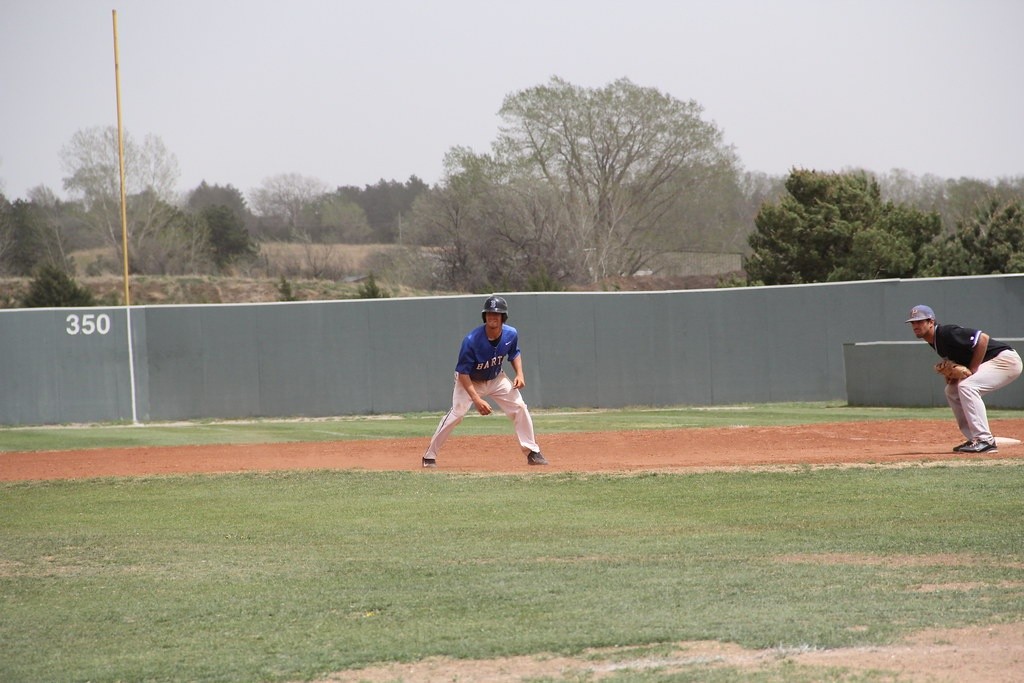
[936,362,973,379]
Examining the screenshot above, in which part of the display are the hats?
[905,306,936,325]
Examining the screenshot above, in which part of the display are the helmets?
[482,296,509,324]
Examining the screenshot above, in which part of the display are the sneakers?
[421,458,436,468]
[528,451,547,465]
[952,438,998,454]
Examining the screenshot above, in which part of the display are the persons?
[422,295,549,468]
[905,304,1023,454]
